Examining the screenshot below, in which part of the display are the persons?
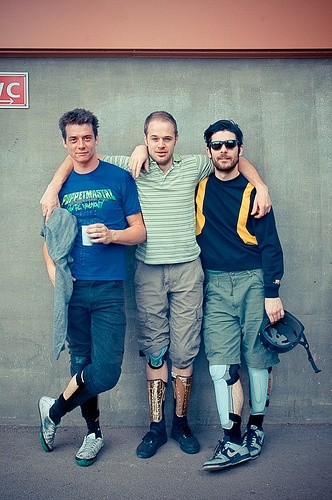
[39,111,271,457]
[188,118,283,471]
[38,109,146,466]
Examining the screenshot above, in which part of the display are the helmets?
[259,311,305,353]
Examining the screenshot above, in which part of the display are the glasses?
[209,140,238,150]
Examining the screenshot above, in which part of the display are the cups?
[81,225,93,246]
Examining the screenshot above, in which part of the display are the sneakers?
[202,438,251,471]
[240,423,265,459]
[170,423,201,454]
[137,430,168,458]
[37,395,62,453]
[75,432,106,467]
